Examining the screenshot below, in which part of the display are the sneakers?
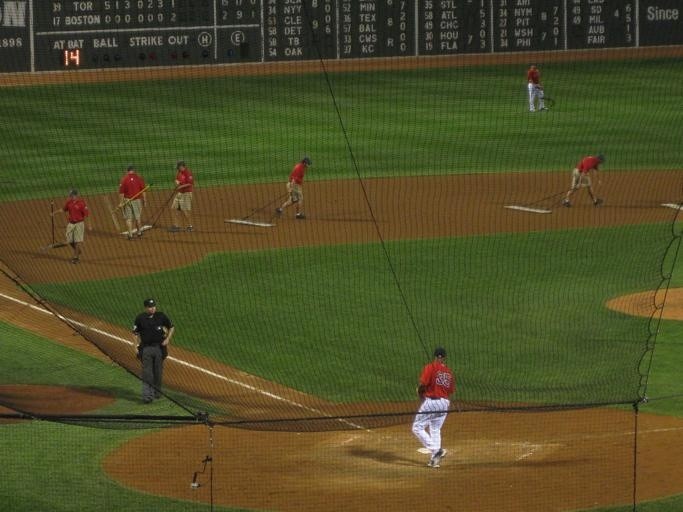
[428,449,446,467]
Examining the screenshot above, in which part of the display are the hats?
[435,349,446,358]
[144,299,155,307]
[305,158,312,165]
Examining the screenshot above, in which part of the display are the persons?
[133,298,175,405]
[412,349,456,468]
[562,154,606,207]
[118,166,147,240]
[50,191,90,263]
[276,158,311,218]
[169,162,194,232]
[527,61,548,112]
